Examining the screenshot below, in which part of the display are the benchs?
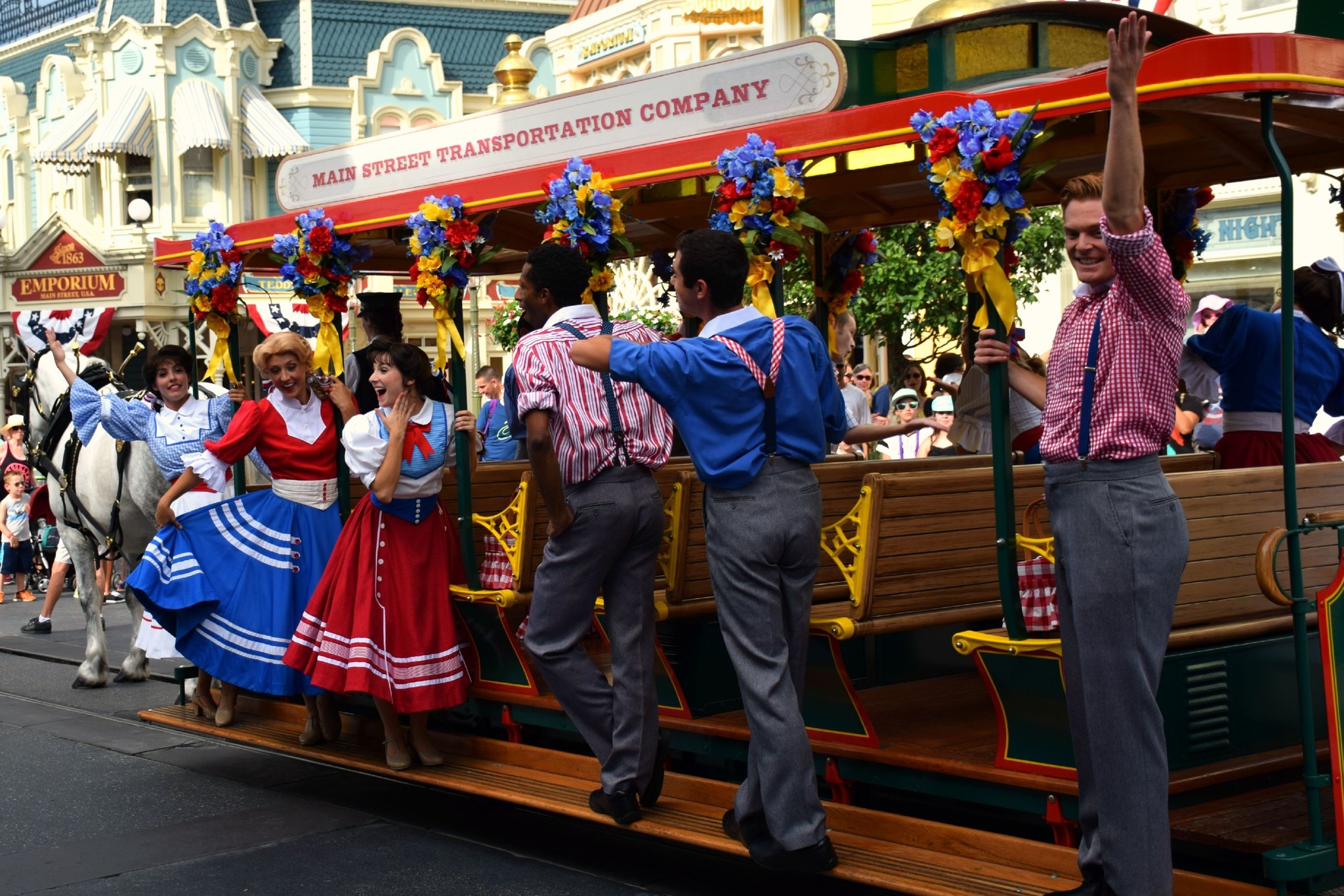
[445,452,863,697]
[953,459,1344,779]
[803,452,1225,751]
[348,462,530,577]
[590,452,1026,721]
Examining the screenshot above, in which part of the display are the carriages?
[10,0,1344,896]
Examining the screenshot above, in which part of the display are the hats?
[931,395,954,412]
[1191,293,1235,329]
[891,388,920,407]
[354,292,402,318]
[1,415,26,432]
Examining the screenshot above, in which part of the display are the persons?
[0,226,1344,875]
[974,13,1192,896]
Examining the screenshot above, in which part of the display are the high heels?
[315,701,341,740]
[218,688,238,726]
[382,737,411,769]
[299,717,323,744]
[191,688,218,720]
[407,731,443,766]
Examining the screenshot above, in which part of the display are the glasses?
[903,373,921,380]
[895,401,919,411]
[855,375,873,381]
[1203,311,1223,320]
[5,413,13,416]
[11,427,23,430]
[7,481,26,487]
[844,373,852,378]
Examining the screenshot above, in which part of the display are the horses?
[14,333,174,688]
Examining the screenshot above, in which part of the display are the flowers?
[709,134,880,317]
[1148,186,1213,283]
[270,209,372,317]
[406,193,479,306]
[185,221,248,323]
[540,158,637,301]
[491,297,522,351]
[610,302,681,333]
[907,97,1059,250]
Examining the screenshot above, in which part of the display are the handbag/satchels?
[1001,555,1061,632]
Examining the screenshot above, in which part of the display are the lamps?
[128,198,151,226]
[811,12,830,35]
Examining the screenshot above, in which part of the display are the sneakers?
[117,581,126,592]
[3,579,14,584]
[588,733,838,872]
[20,617,51,634]
[13,591,36,602]
[104,591,126,603]
[1044,877,1113,896]
[100,617,105,630]
[0,592,5,604]
